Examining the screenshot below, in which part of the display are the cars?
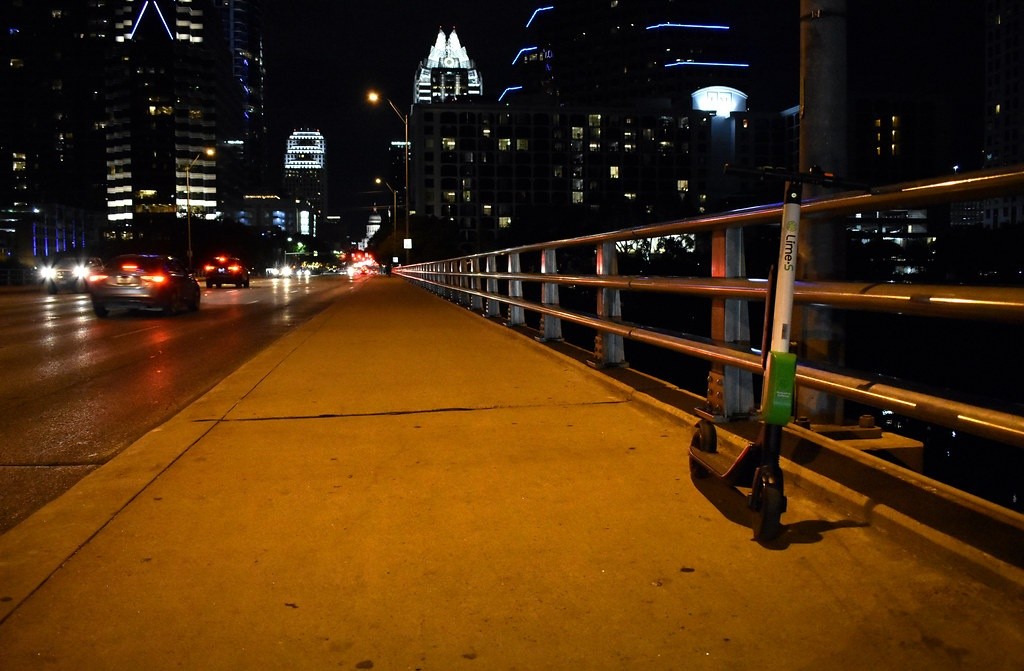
[91,253,201,318]
[204,257,251,289]
[46,256,105,292]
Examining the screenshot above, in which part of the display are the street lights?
[186,148,215,271]
[367,92,409,264]
[374,175,397,258]
